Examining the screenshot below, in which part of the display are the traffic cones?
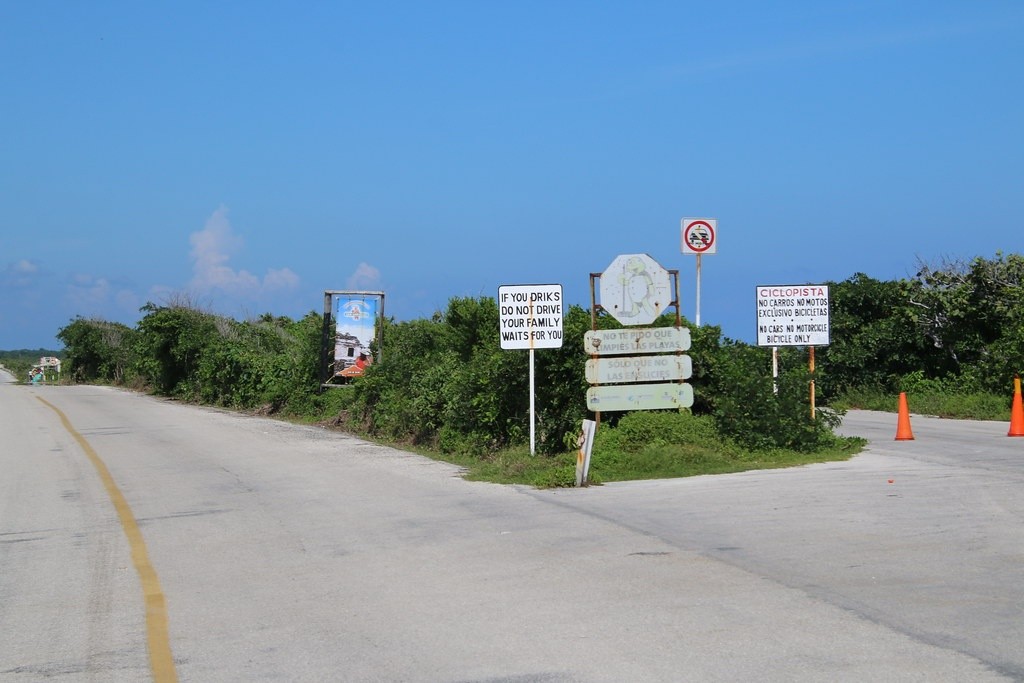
[1008,374,1024,436]
[895,392,915,440]
